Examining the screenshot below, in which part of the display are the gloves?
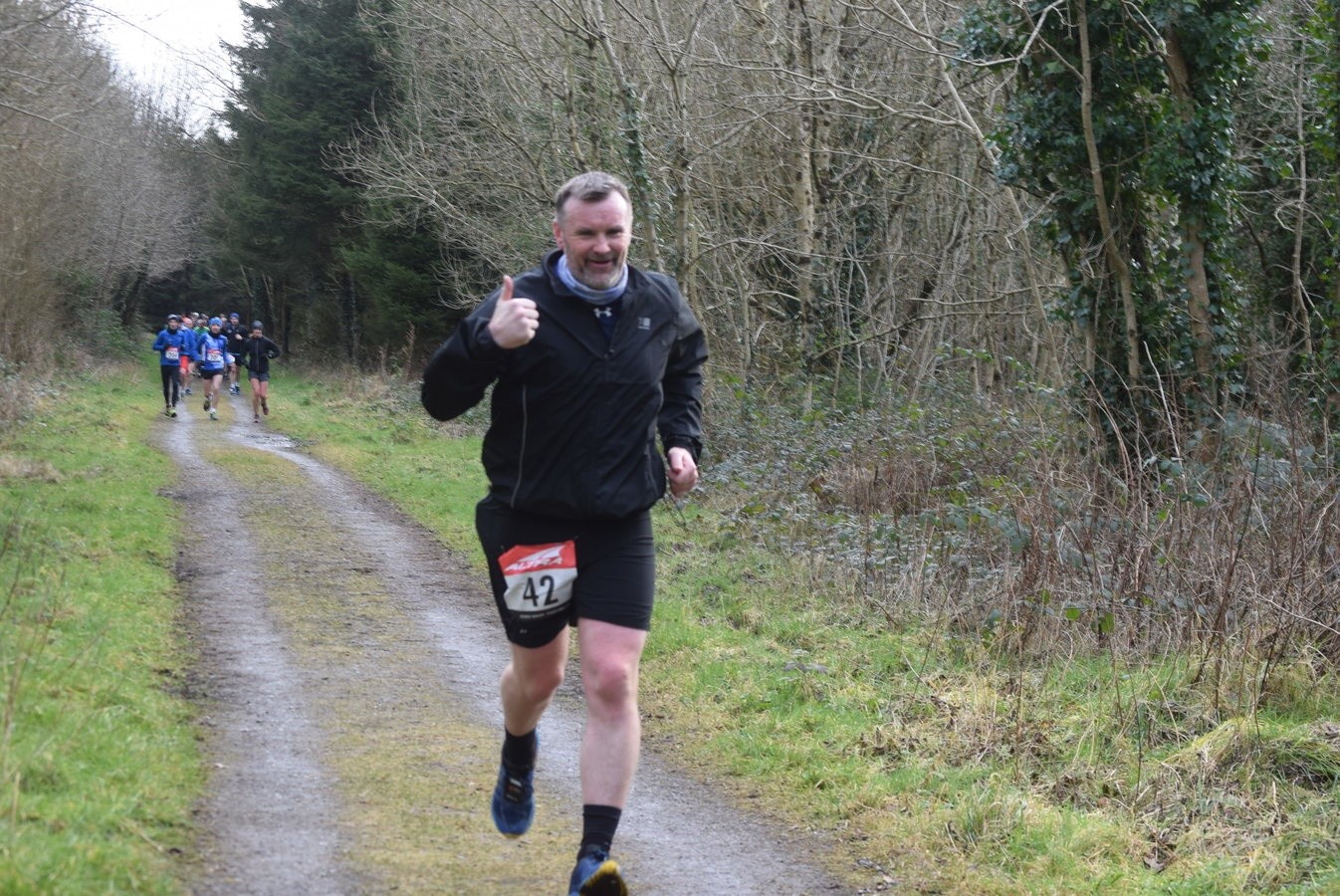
[268,353,275,358]
[200,362,206,367]
[235,356,243,366]
[226,363,231,372]
[164,340,173,348]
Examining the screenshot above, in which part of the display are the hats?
[252,320,263,329]
[167,314,179,321]
[229,313,239,318]
[209,318,222,328]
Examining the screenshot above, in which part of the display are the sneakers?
[261,403,269,415]
[210,412,218,420]
[253,414,259,423]
[234,385,240,395]
[166,407,177,418]
[491,727,539,838]
[230,387,236,394]
[569,857,629,896]
[204,399,210,411]
[186,389,191,395]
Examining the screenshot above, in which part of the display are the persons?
[241,320,279,427]
[419,169,711,895]
[152,314,189,419]
[194,318,236,421]
[164,312,248,399]
